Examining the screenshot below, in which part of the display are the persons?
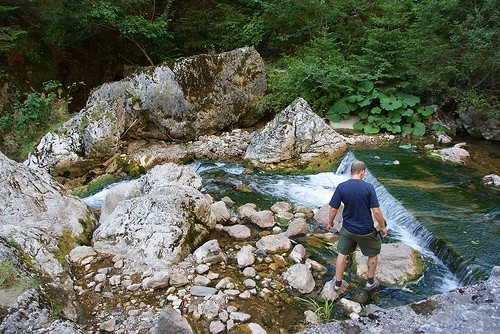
[326,160,387,293]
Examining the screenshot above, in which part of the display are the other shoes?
[333,282,343,292]
[365,278,379,290]
